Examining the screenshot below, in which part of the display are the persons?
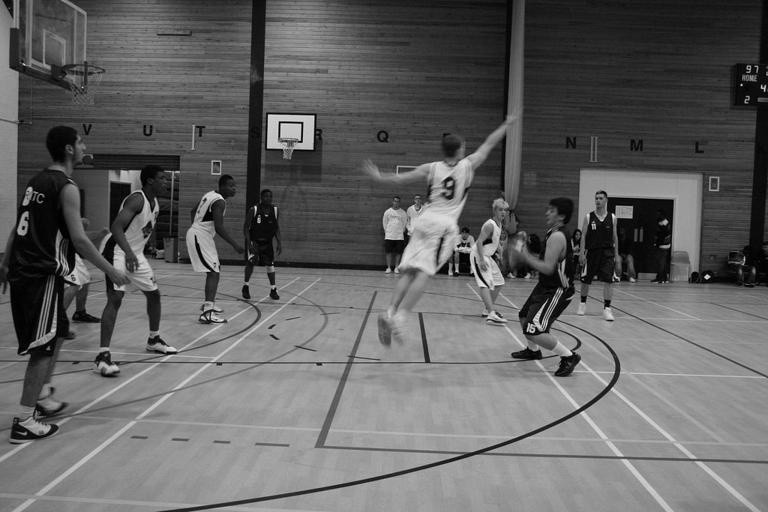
[241,186,284,302]
[1,123,130,447]
[182,175,247,325]
[360,106,523,351]
[55,217,105,340]
[94,161,178,378]
[735,243,756,288]
[381,192,676,284]
[577,189,628,323]
[508,196,589,377]
[755,240,768,287]
[467,197,510,327]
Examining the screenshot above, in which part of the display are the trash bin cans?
[163,236,178,263]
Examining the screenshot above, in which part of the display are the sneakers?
[146,334,177,354]
[469,272,474,276]
[242,285,250,299]
[511,347,543,360]
[506,271,539,279]
[385,266,399,274]
[448,270,460,276]
[602,307,615,321]
[578,302,586,315]
[10,410,60,442]
[481,308,508,325]
[270,287,279,299]
[93,350,121,376]
[554,350,580,377]
[630,277,636,283]
[199,306,226,324]
[72,311,100,322]
[377,310,407,348]
[614,277,620,282]
[651,277,669,284]
[35,386,68,419]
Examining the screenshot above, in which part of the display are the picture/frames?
[211,160,222,176]
[709,176,720,192]
